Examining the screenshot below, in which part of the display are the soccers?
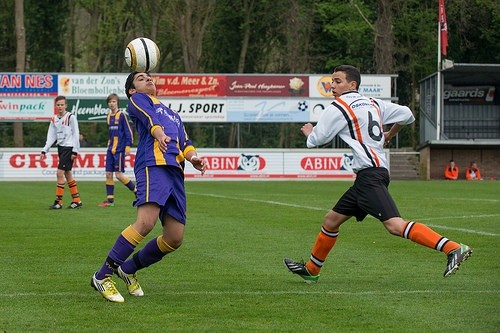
[124,36,161,73]
